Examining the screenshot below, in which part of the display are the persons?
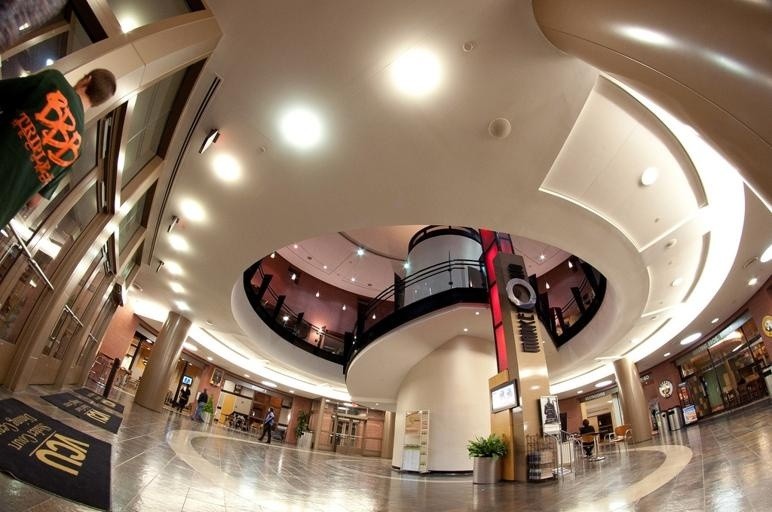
[579,419,595,458]
[257,407,275,443]
[191,388,208,423]
[176,384,190,413]
[0,68,117,230]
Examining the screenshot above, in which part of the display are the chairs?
[578,423,636,460]
[726,380,765,408]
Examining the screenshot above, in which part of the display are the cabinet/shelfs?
[525,430,578,483]
[399,408,430,476]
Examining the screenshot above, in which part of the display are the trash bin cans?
[654,405,684,434]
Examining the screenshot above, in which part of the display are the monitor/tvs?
[182,375,193,386]
[490,379,518,414]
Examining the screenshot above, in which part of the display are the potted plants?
[201,393,215,424]
[464,431,509,485]
[294,408,314,449]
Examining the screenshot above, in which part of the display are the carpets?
[0,387,125,512]
